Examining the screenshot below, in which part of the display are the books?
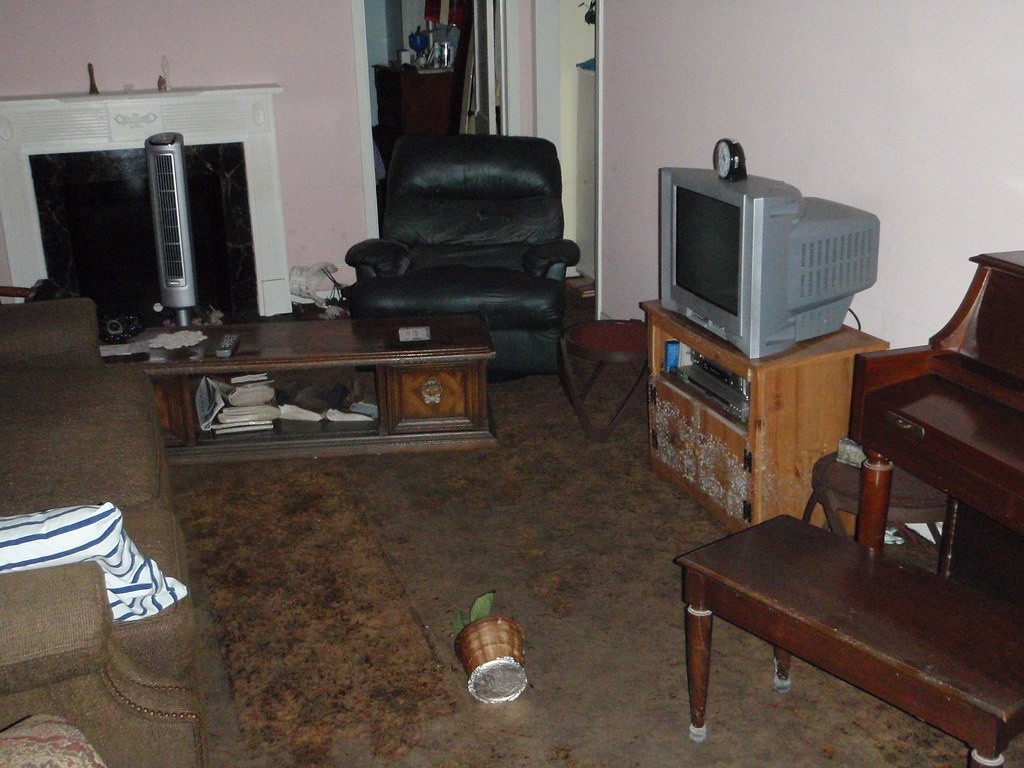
[195,373,281,435]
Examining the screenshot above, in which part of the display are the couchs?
[0,301,206,768]
[342,131,582,386]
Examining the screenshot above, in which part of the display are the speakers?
[146,130,199,328]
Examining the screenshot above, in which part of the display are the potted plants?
[452,594,529,702]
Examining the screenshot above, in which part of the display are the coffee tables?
[106,312,501,465]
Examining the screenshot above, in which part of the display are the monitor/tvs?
[658,168,880,359]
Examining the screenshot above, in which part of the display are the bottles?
[433,42,440,69]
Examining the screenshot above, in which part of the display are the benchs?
[673,514,1024,768]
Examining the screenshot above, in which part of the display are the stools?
[801,448,947,551]
[551,320,650,445]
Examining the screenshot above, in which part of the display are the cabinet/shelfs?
[632,299,889,537]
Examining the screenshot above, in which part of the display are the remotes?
[215,334,240,358]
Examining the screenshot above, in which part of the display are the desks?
[370,64,464,137]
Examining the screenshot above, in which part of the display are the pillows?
[0,712,112,768]
[0,502,189,624]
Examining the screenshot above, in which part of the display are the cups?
[441,43,450,67]
